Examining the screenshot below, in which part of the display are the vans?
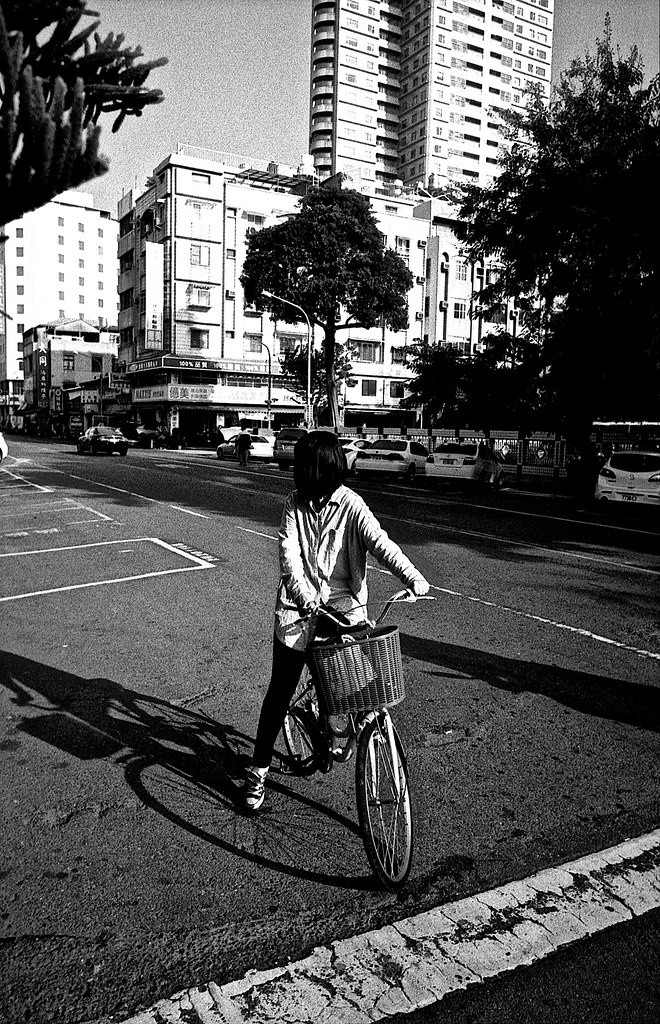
[274,427,329,469]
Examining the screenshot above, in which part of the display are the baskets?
[304,624,406,717]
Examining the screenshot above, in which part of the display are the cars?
[216,434,273,464]
[596,451,660,507]
[335,436,373,470]
[424,442,505,483]
[77,425,130,456]
[355,439,429,477]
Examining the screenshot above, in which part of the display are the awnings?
[177,404,305,414]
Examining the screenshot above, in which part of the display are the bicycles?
[281,584,439,894]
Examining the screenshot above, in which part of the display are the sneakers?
[245,766,267,811]
[358,710,387,746]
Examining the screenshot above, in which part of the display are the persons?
[235,425,252,467]
[241,433,430,815]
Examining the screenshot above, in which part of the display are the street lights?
[261,289,311,405]
